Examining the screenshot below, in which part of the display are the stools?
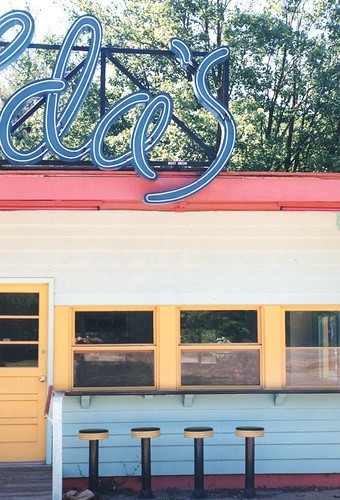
[130,426,162,500]
[183,426,214,500]
[77,428,111,500]
[235,426,267,500]
